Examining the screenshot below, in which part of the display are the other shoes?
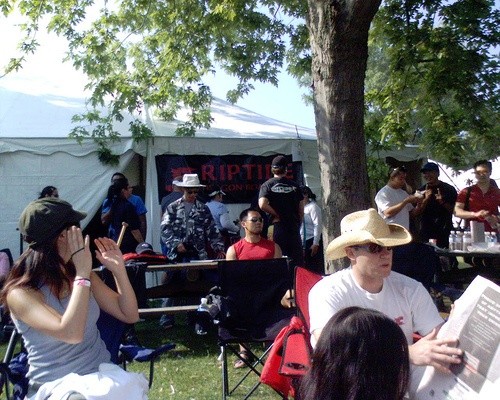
[159,319,174,328]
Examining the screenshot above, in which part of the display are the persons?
[0,197,139,400]
[39,186,58,198]
[300,306,410,400]
[101,172,147,322]
[309,208,462,375]
[374,161,500,295]
[160,156,323,368]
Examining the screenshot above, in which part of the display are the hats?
[271,156,287,168]
[209,189,226,196]
[136,242,154,254]
[420,162,439,172]
[173,173,206,186]
[18,197,87,242]
[326,208,412,259]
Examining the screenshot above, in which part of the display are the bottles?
[449,231,497,252]
[196,298,209,335]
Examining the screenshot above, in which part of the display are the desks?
[429,242,500,257]
[147,261,218,271]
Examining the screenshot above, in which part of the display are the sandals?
[235,352,253,369]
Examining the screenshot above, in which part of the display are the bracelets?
[74,276,91,286]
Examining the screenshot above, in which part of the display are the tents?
[1,86,429,260]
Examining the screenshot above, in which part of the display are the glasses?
[352,244,393,252]
[246,217,263,223]
[393,165,404,171]
[184,190,199,193]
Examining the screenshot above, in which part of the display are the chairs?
[196,257,295,400]
[0,247,175,400]
[260,266,424,400]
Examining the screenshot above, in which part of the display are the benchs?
[138,305,199,315]
